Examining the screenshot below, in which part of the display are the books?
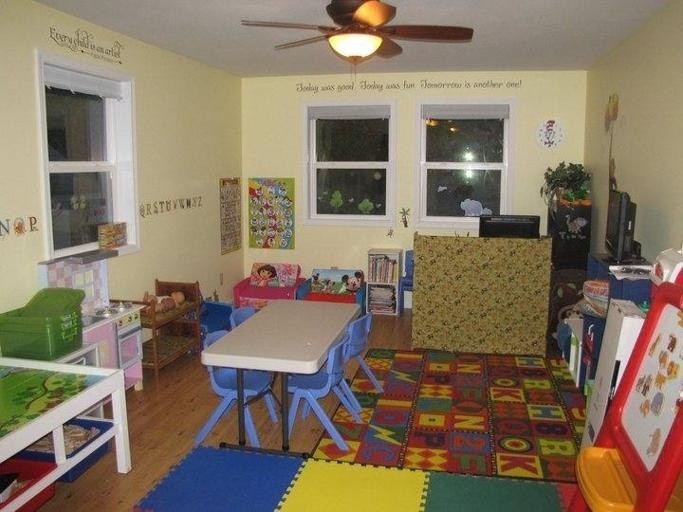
[368,252,396,314]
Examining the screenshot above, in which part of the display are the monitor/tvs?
[604,188,636,264]
[478,213,540,236]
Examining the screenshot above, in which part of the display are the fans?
[240,0,473,58]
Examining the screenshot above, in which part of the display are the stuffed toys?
[140,298,176,316]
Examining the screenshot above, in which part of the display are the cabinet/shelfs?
[55,303,144,392]
[578,252,653,397]
[367,249,404,318]
[109,278,201,376]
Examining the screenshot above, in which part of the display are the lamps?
[328,33,383,65]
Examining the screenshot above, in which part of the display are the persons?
[144,290,185,304]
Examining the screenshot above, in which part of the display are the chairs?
[202,276,385,452]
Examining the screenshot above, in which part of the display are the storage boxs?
[0,458,58,512]
[0,287,85,362]
[15,418,114,482]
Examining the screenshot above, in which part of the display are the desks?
[0,356,133,512]
[399,276,413,313]
